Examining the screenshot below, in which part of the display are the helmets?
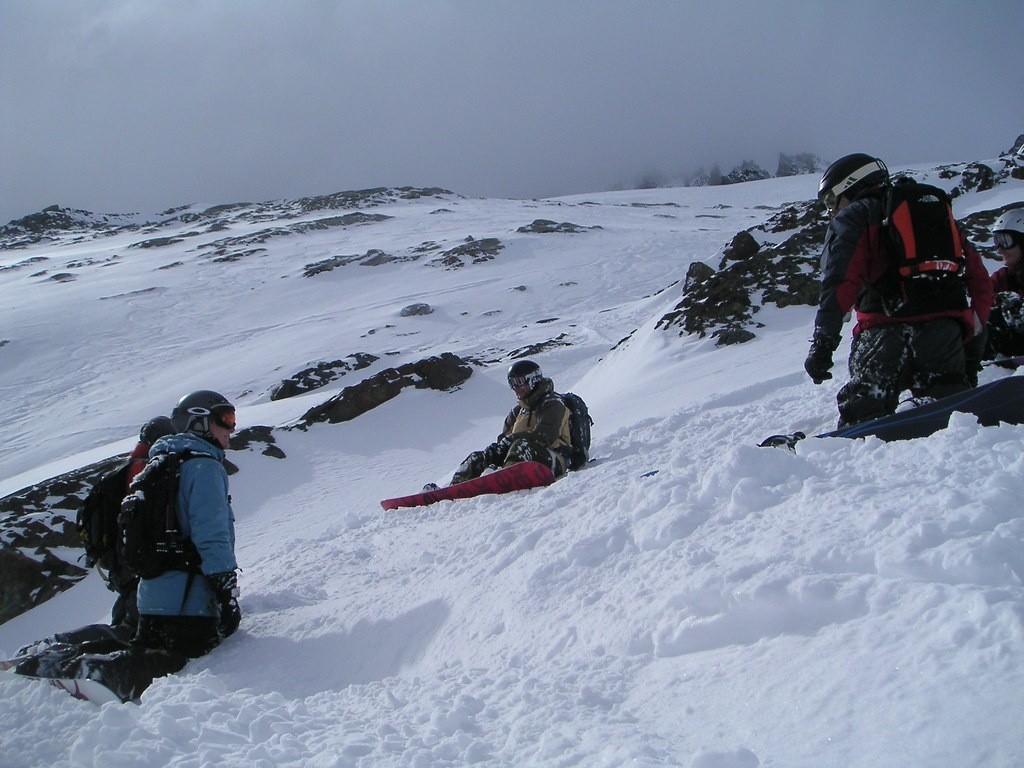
[139,416,179,446]
[990,208,1024,234]
[818,154,890,204]
[171,390,236,433]
[507,359,542,392]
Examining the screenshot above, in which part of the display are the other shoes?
[423,483,440,491]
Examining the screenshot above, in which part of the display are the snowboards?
[785,375,1024,451]
[380,461,557,513]
[17,674,123,707]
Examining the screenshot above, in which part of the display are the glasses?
[217,410,236,429]
[509,377,526,390]
[993,233,1016,250]
[820,188,842,218]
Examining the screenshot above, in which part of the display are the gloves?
[996,291,1024,329]
[804,324,842,385]
[482,443,508,471]
[219,586,241,638]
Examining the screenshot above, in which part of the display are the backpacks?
[75,463,133,570]
[855,176,969,317]
[553,391,594,471]
[116,448,220,578]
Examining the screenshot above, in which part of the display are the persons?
[803,154,994,431]
[984,208,1024,361]
[422,361,572,493]
[16,390,242,705]
